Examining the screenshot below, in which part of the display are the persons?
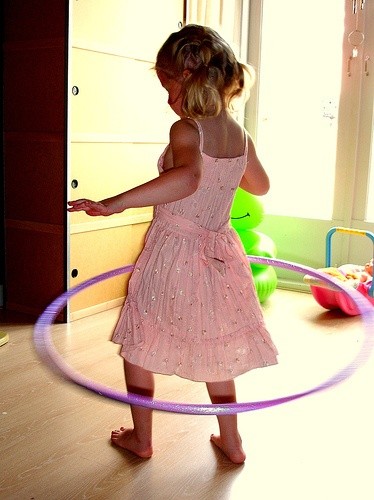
[65,24,270,463]
